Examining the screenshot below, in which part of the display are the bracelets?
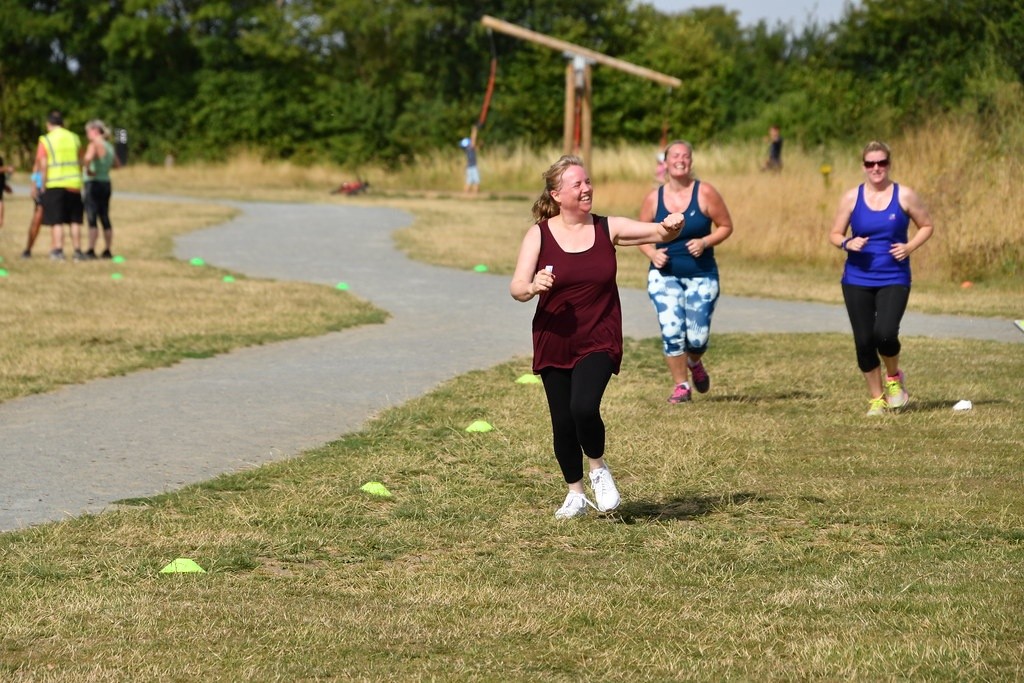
[702,238,708,249]
[841,237,854,251]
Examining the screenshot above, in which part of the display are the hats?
[461,138,472,148]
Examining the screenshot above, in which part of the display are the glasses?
[864,158,887,167]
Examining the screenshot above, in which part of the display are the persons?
[762,126,784,173]
[0,108,128,259]
[639,140,734,403]
[461,126,481,195]
[828,140,935,419]
[510,155,685,519]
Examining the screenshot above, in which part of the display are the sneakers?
[667,382,691,404]
[687,359,710,393]
[885,369,909,407]
[865,391,889,417]
[588,460,621,512]
[555,489,599,519]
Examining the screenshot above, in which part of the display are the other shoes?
[86,249,96,258]
[101,249,113,258]
[73,249,83,258]
[21,250,31,257]
[50,248,63,258]
